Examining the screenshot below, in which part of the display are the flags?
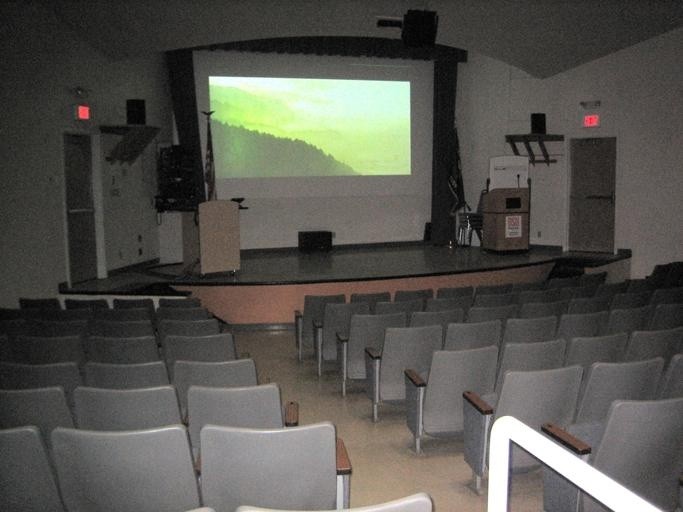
[446,124,464,215]
[205,120,216,200]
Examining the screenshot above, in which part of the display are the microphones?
[517,174,521,188]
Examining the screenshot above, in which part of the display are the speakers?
[127,99,146,124]
[531,113,546,135]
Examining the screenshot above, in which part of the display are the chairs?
[455,186,489,248]
[1,257,683,512]
